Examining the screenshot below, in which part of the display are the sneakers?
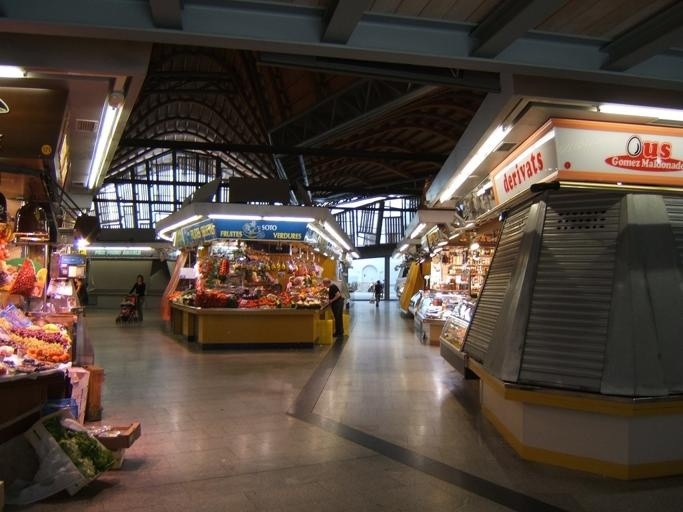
[333,331,346,337]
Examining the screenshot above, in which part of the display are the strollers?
[116,294,139,322]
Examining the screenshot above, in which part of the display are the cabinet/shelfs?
[1,249,85,512]
[395,229,501,381]
[159,239,349,348]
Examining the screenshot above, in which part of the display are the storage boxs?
[23,364,142,497]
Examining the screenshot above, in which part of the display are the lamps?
[80,90,128,193]
[14,172,99,251]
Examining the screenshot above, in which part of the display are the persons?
[72,277,88,318]
[320,278,345,337]
[130,274,146,320]
[373,280,384,306]
